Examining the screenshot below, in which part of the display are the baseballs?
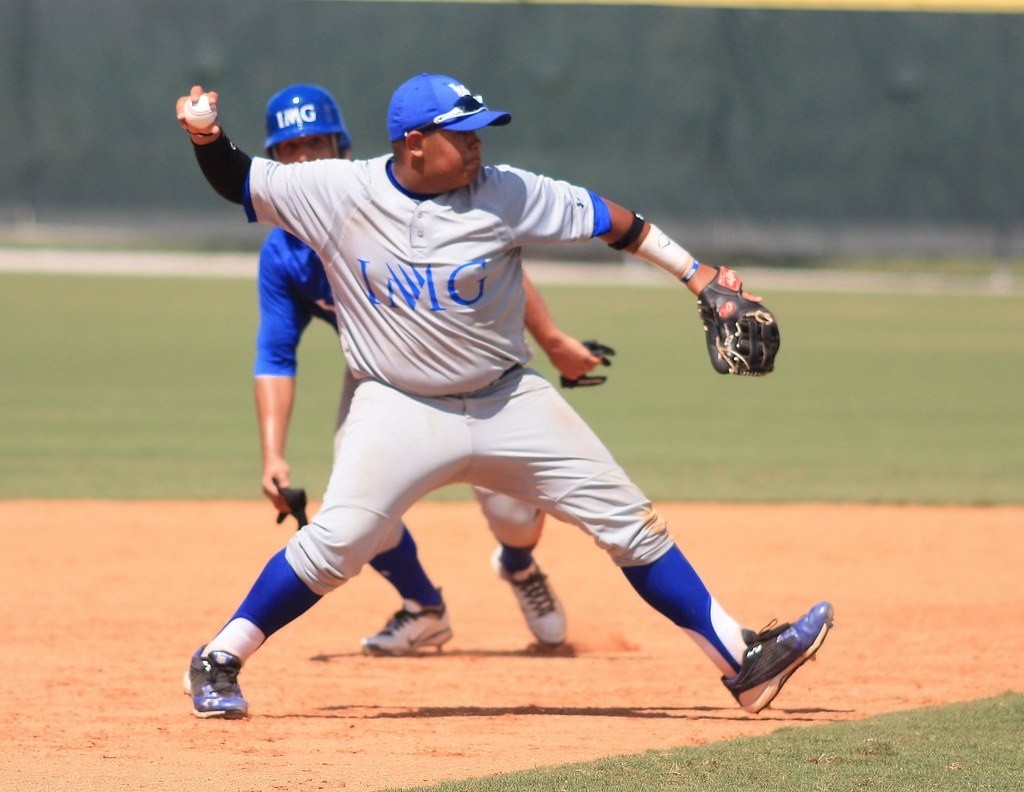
[181,94,218,130]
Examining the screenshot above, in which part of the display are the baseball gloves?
[695,265,781,378]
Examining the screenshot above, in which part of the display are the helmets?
[264,84,352,159]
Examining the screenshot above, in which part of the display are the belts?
[492,363,521,385]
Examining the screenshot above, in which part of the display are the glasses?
[404,94,487,138]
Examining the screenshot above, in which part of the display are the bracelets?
[682,261,699,282]
[188,130,213,137]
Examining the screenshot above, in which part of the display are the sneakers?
[360,587,453,656]
[720,601,835,715]
[492,548,567,645]
[183,645,249,718]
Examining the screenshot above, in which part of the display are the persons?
[175,73,835,718]
[252,84,601,658]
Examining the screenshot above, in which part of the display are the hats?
[387,72,512,142]
